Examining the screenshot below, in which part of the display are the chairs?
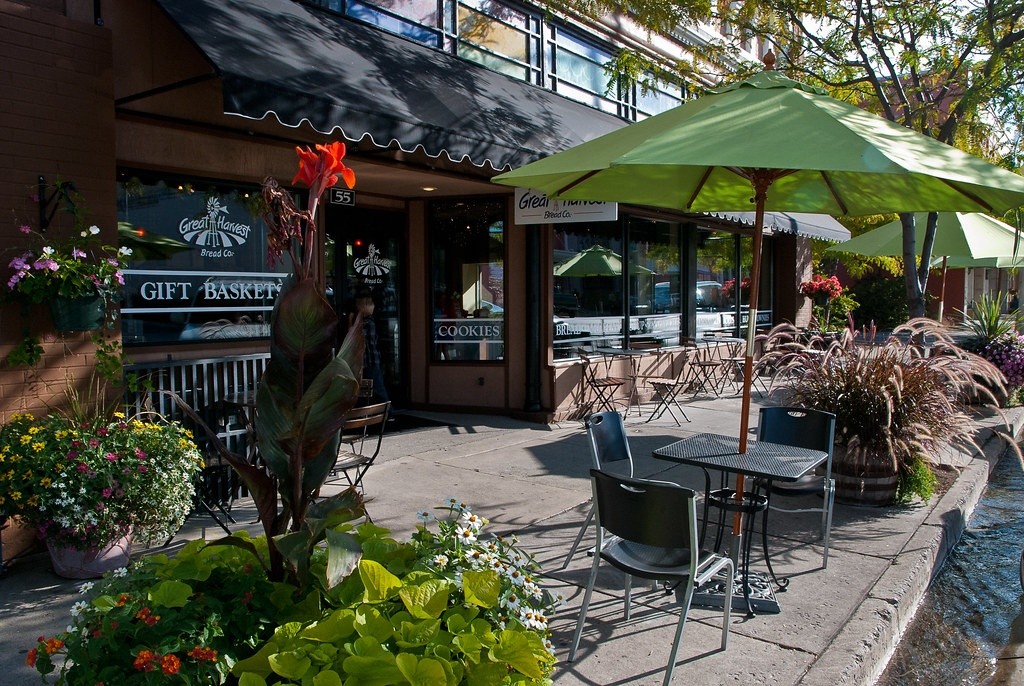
[161,400,258,550]
[563,411,660,592]
[742,407,837,567]
[564,468,734,686]
[307,377,390,526]
[576,329,842,426]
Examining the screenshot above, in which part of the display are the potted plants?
[751,311,1024,506]
[949,295,1024,407]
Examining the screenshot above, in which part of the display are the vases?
[815,295,829,306]
[39,282,104,334]
[36,518,134,578]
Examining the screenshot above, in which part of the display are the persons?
[1008,288,1019,314]
[354,294,396,422]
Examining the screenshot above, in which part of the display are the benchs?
[118,349,272,500]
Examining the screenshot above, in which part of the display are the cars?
[481,300,503,314]
[552,286,582,320]
[638,281,749,315]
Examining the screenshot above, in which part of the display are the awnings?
[159,0,851,243]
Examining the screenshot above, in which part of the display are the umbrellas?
[553,244,652,316]
[488,48,1024,584]
[825,212,1024,340]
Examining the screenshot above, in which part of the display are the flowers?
[6,191,156,393]
[798,271,842,302]
[967,333,1024,397]
[1,372,205,559]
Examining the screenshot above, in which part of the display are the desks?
[653,432,828,619]
[701,337,744,393]
[595,348,651,419]
